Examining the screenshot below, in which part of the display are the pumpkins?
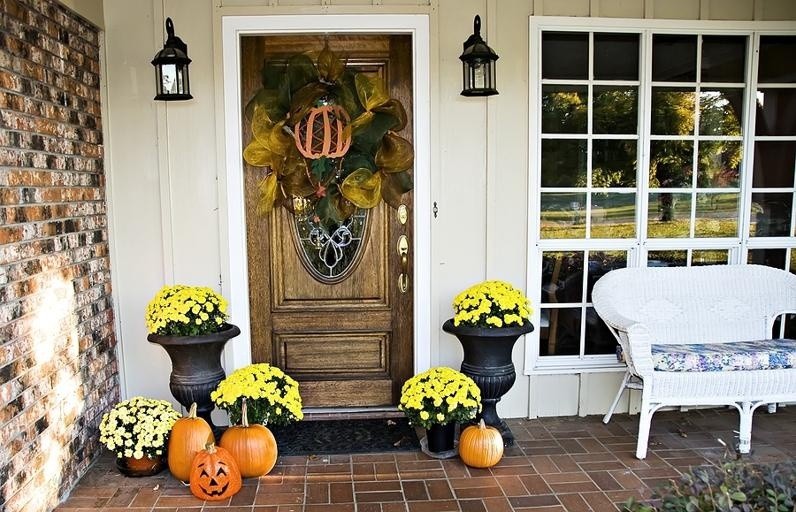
[458,418,504,468]
[167,402,216,482]
[217,395,277,477]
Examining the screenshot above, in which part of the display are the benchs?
[591,262,796,461]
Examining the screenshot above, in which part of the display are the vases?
[425,420,457,456]
[146,325,241,429]
[119,443,158,471]
[440,319,537,451]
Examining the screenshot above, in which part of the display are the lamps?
[150,16,193,103]
[458,14,497,98]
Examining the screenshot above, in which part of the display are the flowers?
[446,280,534,328]
[207,364,304,432]
[143,282,226,336]
[400,369,483,429]
[92,397,182,460]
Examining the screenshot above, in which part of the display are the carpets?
[270,415,421,458]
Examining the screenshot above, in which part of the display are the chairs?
[538,221,568,355]
